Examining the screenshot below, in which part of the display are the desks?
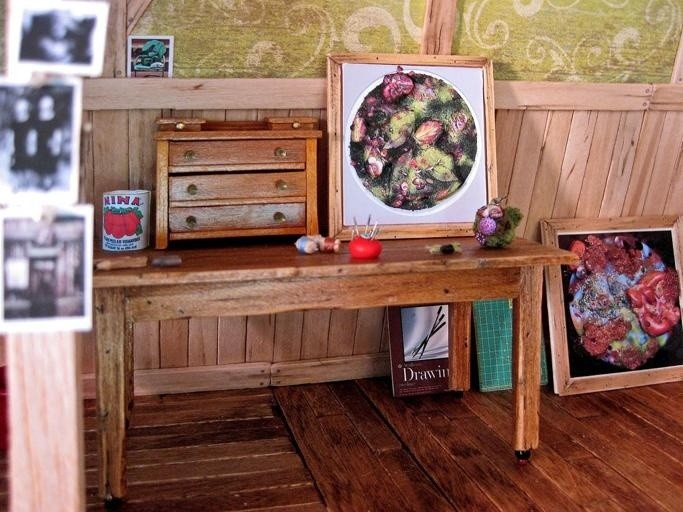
[94,232,581,505]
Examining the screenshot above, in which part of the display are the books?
[386,300,548,397]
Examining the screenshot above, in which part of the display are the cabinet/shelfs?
[154,114,324,250]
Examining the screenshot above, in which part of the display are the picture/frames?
[126,33,176,76]
[328,52,497,243]
[540,214,683,399]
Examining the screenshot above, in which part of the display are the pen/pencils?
[351,214,383,241]
